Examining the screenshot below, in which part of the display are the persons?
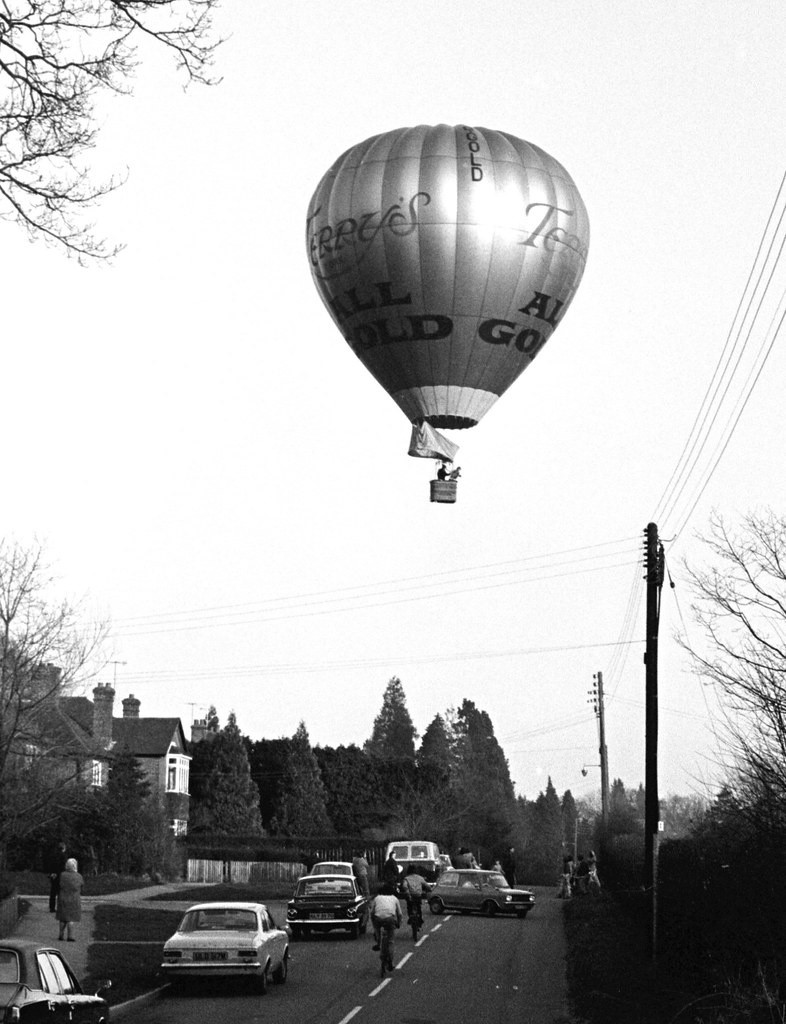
[450,467,461,479]
[502,847,515,889]
[555,848,601,899]
[354,850,369,899]
[383,851,438,896]
[492,860,504,875]
[370,882,402,970]
[438,465,450,481]
[403,865,431,927]
[49,842,67,913]
[56,857,84,942]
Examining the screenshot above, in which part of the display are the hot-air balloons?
[305,121,592,507]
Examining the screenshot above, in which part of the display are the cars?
[0,940,117,1024]
[428,868,537,917]
[287,873,371,939]
[309,860,357,878]
[159,902,291,993]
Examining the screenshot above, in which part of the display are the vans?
[385,840,441,892]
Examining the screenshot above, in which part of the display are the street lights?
[581,764,610,858]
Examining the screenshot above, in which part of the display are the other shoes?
[373,945,380,951]
[407,919,411,925]
[67,938,75,941]
[50,910,56,912]
[387,959,394,972]
[58,937,64,941]
